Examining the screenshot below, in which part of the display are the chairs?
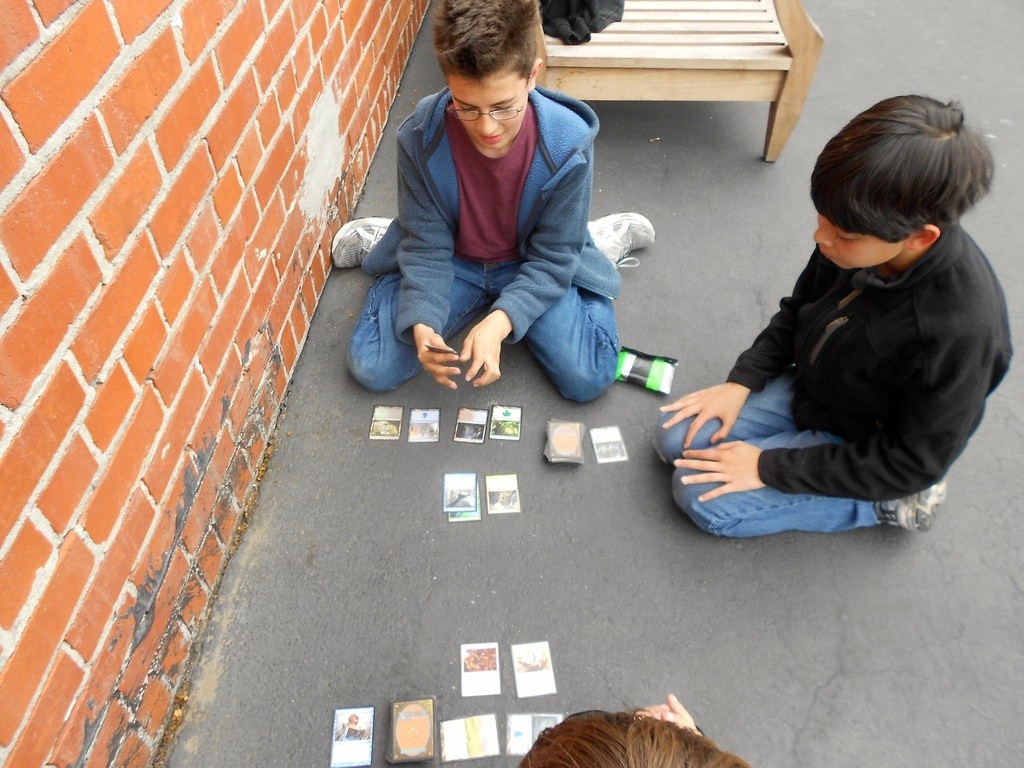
[535,0,826,162]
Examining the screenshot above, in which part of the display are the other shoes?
[875,477,946,534]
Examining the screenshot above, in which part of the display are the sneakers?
[331,216,394,269]
[588,212,656,268]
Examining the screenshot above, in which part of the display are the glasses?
[446,80,528,121]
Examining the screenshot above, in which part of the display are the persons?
[517,693,752,768]
[653,94,1013,538]
[329,0,655,403]
